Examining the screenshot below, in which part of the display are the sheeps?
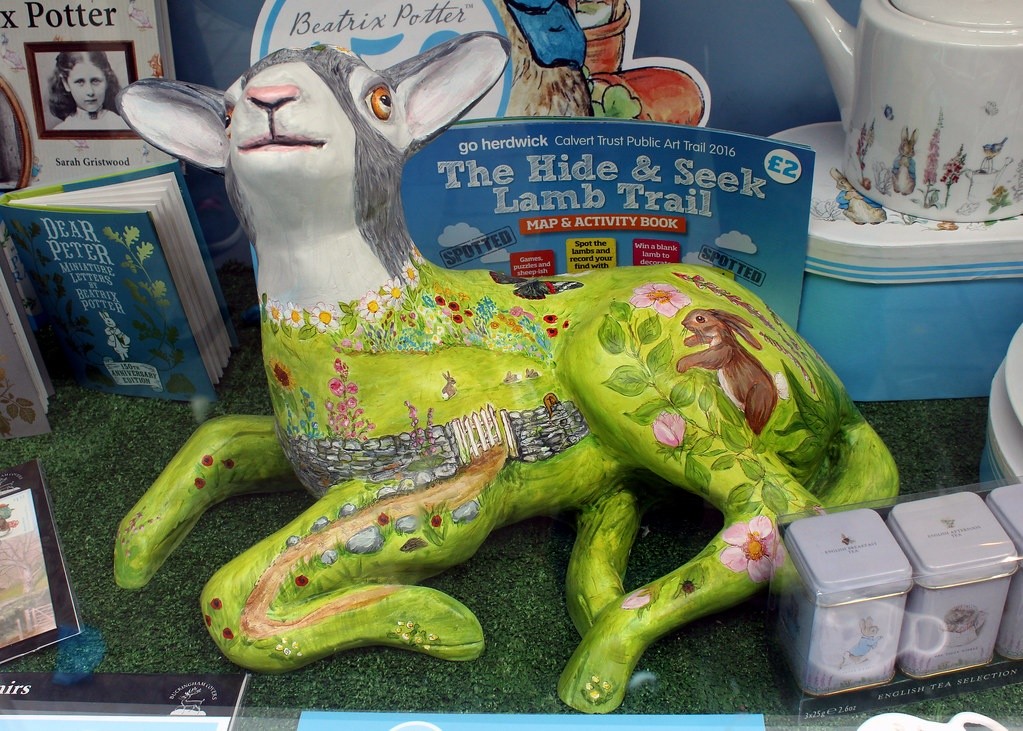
[113,32,900,716]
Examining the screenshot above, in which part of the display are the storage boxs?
[777,484,1023,696]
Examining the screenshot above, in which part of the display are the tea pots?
[784,0,1023,223]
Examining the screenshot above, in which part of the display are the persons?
[48,51,132,130]
